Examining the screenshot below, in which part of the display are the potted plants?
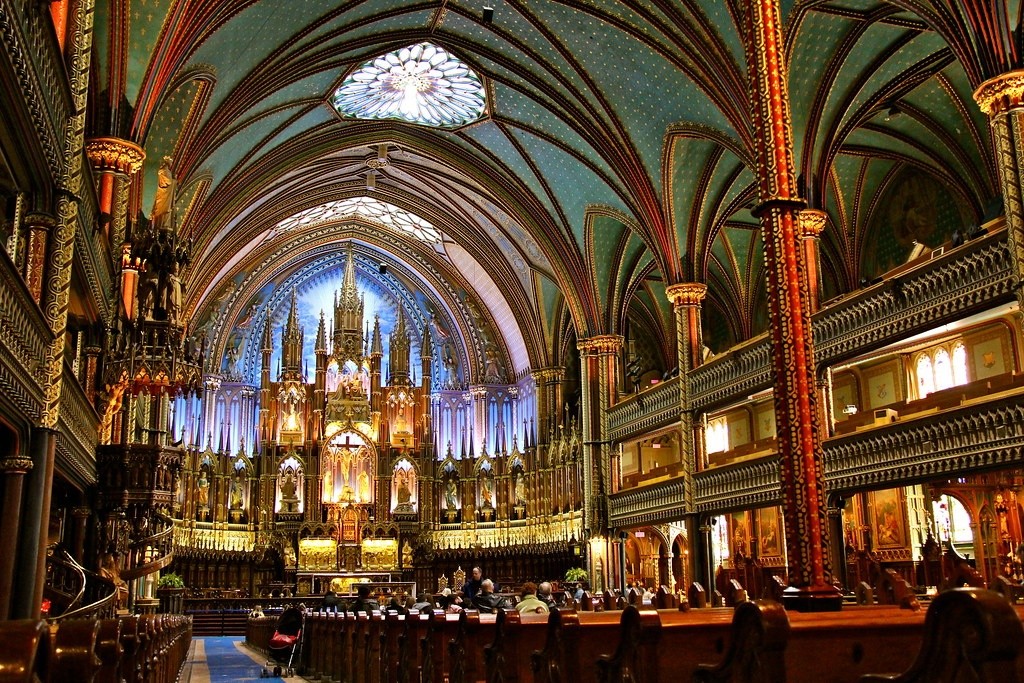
[157,570,186,589]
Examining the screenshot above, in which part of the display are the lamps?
[378,145,388,163]
[367,170,375,191]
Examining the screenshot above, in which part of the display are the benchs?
[0,613,193,683]
[245,573,1024,683]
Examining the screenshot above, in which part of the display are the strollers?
[260,606,307,679]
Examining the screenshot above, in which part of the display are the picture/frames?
[730,506,782,559]
[842,488,906,551]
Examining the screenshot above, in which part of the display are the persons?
[537,582,557,611]
[231,477,243,510]
[515,581,549,614]
[436,567,512,615]
[359,471,368,501]
[482,477,492,506]
[332,375,360,400]
[334,444,362,486]
[445,479,457,509]
[249,604,265,618]
[397,408,406,433]
[282,404,301,430]
[325,471,332,501]
[348,588,380,615]
[280,476,296,500]
[626,579,654,604]
[298,602,306,616]
[574,583,584,599]
[398,478,412,504]
[316,592,346,613]
[515,474,525,502]
[198,472,210,505]
[386,594,435,615]
